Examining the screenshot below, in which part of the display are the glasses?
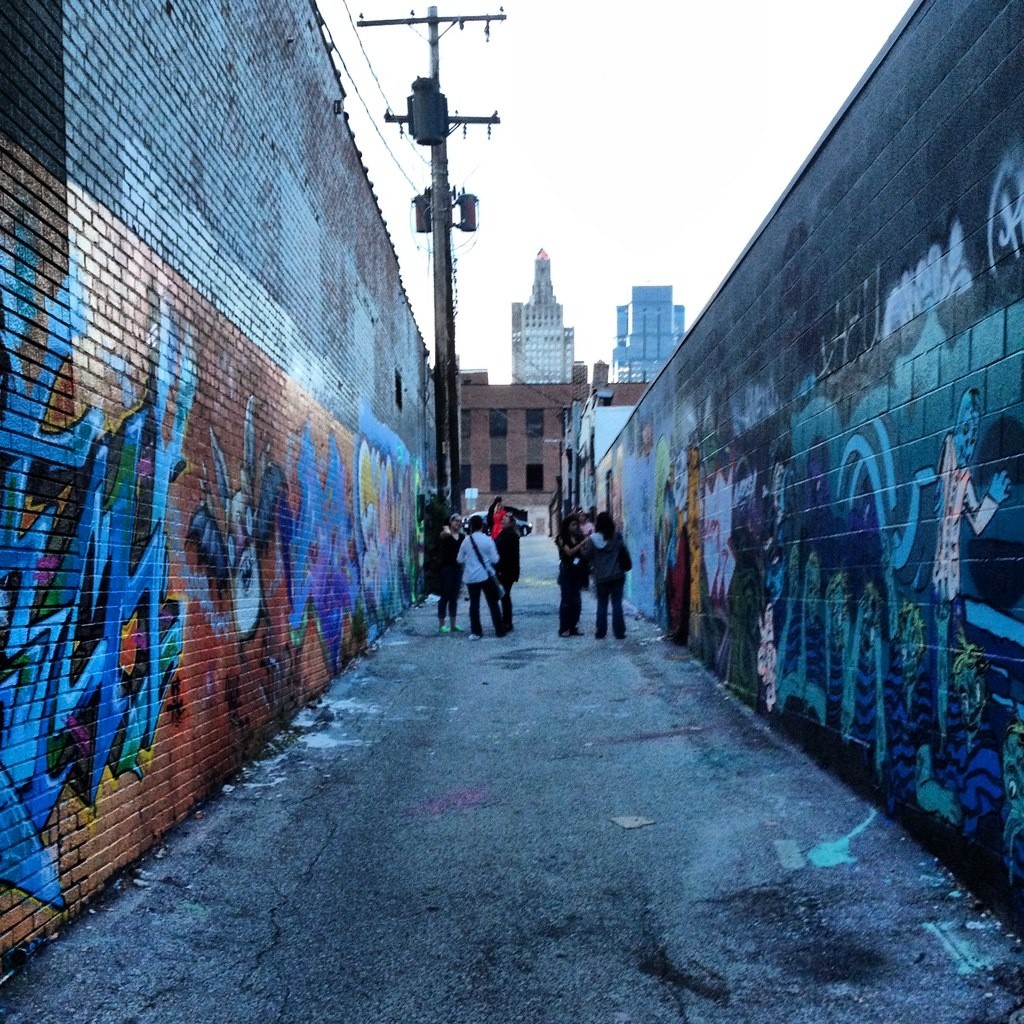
[569,523,578,528]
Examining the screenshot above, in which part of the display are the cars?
[466,510,533,537]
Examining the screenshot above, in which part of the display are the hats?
[450,513,463,524]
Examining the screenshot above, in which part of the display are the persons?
[554,506,628,641]
[435,497,520,640]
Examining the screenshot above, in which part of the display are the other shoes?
[504,623,513,632]
[450,626,465,632]
[559,628,570,637]
[569,628,585,636]
[469,634,479,639]
[438,625,450,632]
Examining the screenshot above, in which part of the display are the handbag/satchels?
[619,544,633,571]
[573,567,589,591]
[489,575,506,602]
